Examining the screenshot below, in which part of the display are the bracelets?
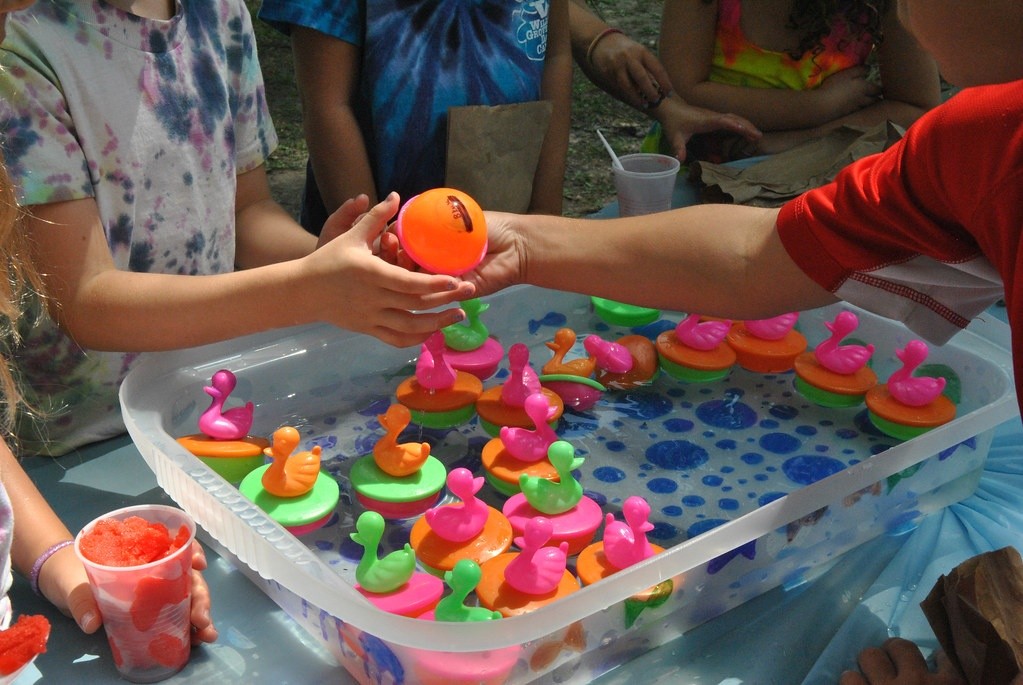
[29,541,78,598]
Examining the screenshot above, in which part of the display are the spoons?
[0,624,51,685]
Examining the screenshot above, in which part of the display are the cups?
[73,504,197,684]
[612,153,681,219]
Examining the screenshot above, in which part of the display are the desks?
[2,153,1023,685]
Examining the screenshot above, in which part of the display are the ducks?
[201,299,947,622]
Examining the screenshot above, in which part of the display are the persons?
[380,2,1023,423]
[300,1,572,245]
[0,2,219,649]
[837,638,962,684]
[0,1,477,458]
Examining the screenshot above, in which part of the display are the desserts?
[0,613,51,677]
[79,517,192,672]
